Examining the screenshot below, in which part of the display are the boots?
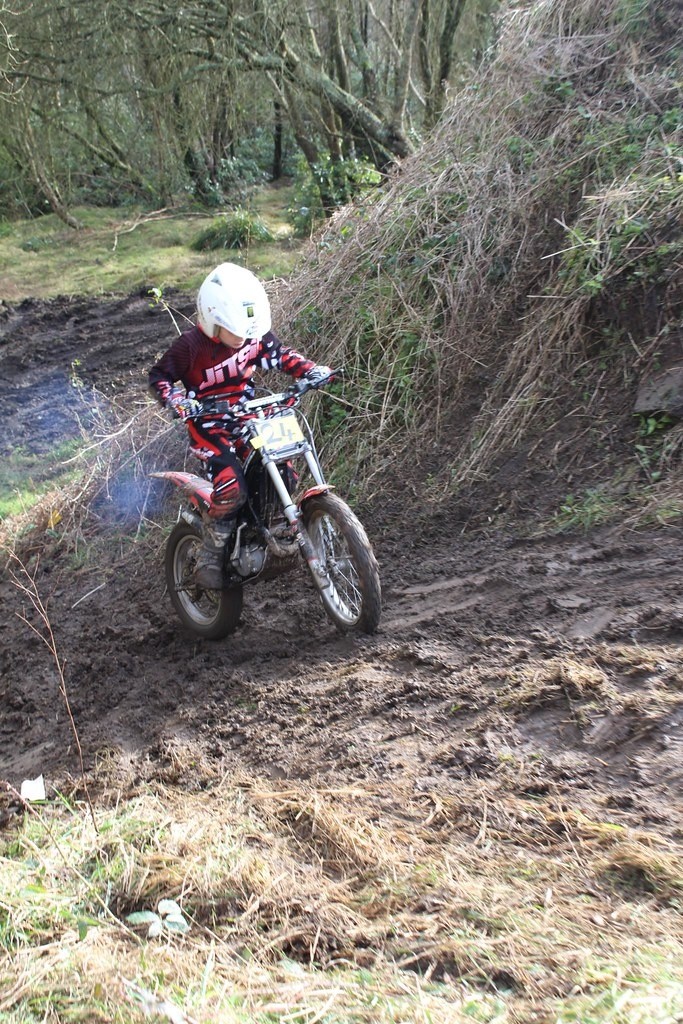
[191,521,231,590]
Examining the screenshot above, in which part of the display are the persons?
[147,264,340,592]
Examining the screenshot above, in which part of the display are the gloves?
[300,364,335,390]
[157,380,203,420]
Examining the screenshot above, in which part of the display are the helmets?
[196,261,272,340]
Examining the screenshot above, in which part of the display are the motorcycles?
[146,368,383,644]
